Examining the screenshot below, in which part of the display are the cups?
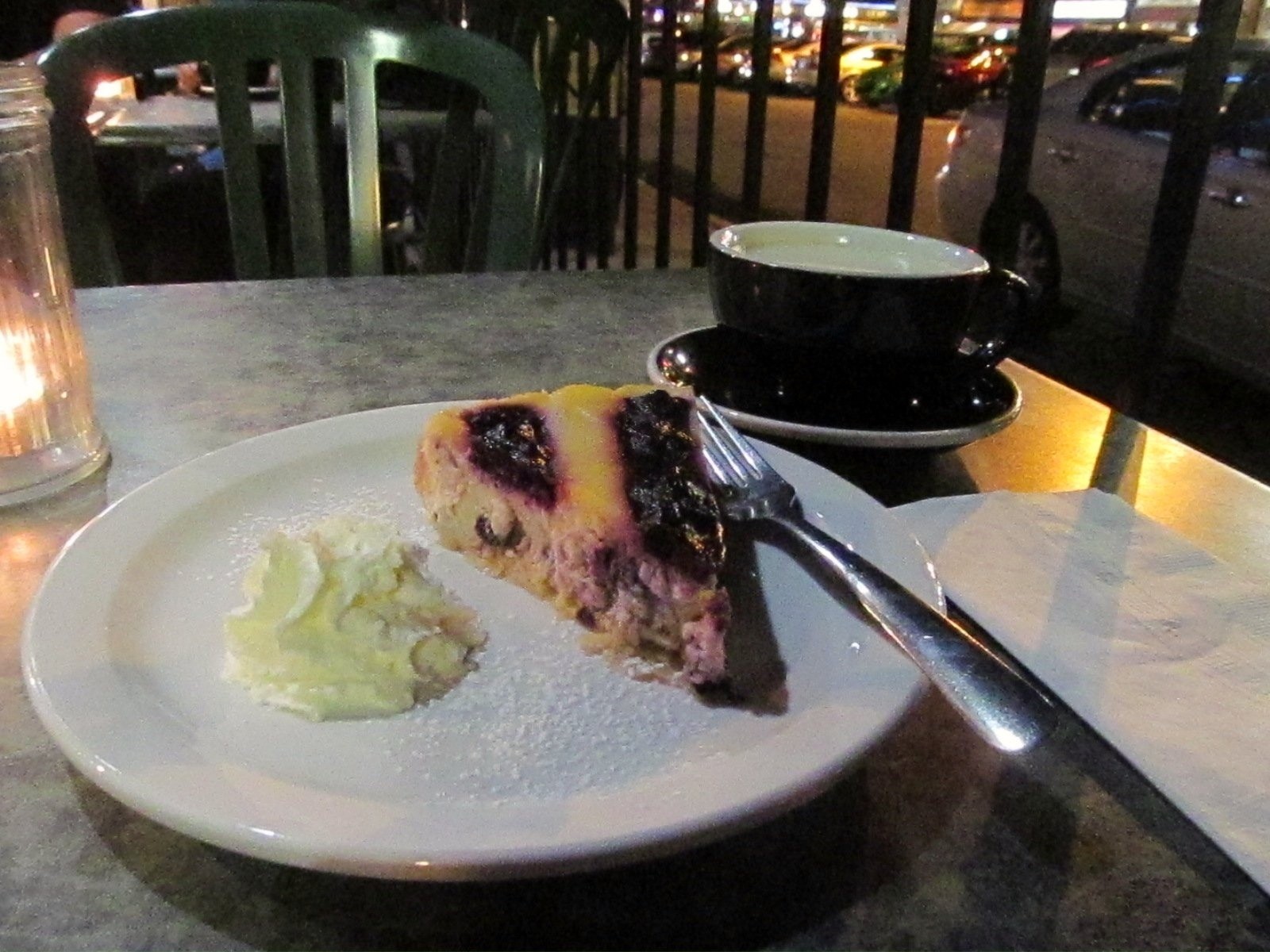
[709,219,1032,430]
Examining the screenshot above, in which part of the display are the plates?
[645,323,1026,470]
[198,85,280,95]
[19,398,947,885]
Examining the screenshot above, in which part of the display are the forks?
[692,393,1061,757]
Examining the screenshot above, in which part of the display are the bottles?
[0,61,112,507]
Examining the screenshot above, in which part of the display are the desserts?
[415,382,728,690]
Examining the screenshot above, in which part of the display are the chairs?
[39,0,629,278]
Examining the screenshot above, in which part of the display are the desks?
[97,90,607,262]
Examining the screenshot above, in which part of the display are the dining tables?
[0,271,1270,952]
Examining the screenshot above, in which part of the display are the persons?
[0,0,416,283]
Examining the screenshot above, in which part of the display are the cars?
[932,25,1269,408]
[641,15,1026,118]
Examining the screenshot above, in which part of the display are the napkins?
[885,486,1270,896]
